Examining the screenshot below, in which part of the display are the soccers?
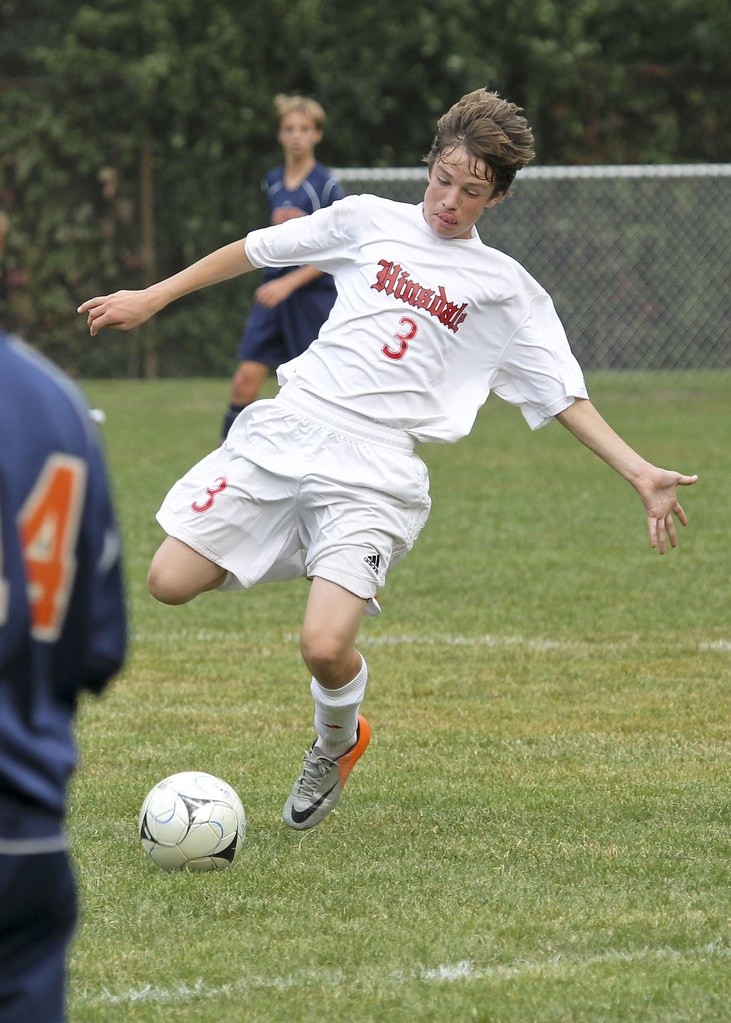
[137,766,246,870]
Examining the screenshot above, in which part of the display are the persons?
[220,93,347,449]
[0,335,129,1023]
[78,89,699,831]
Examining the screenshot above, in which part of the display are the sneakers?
[283,713,370,830]
